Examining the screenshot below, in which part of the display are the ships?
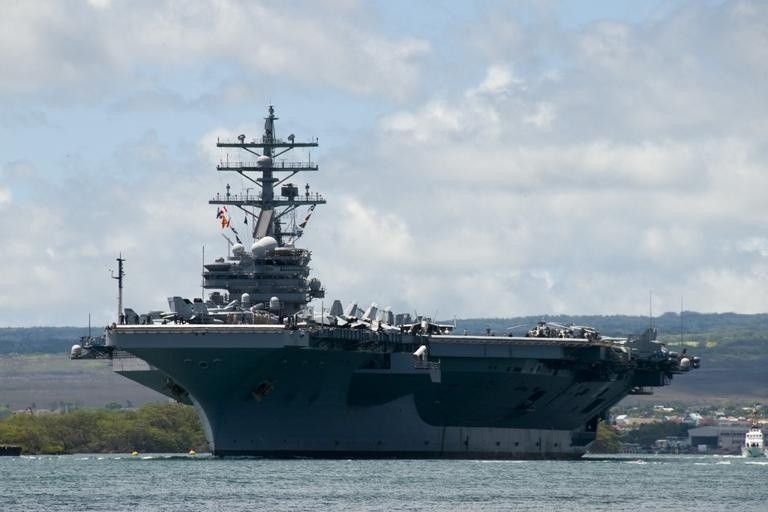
[71,102,702,464]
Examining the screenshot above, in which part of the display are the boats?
[738,421,766,463]
